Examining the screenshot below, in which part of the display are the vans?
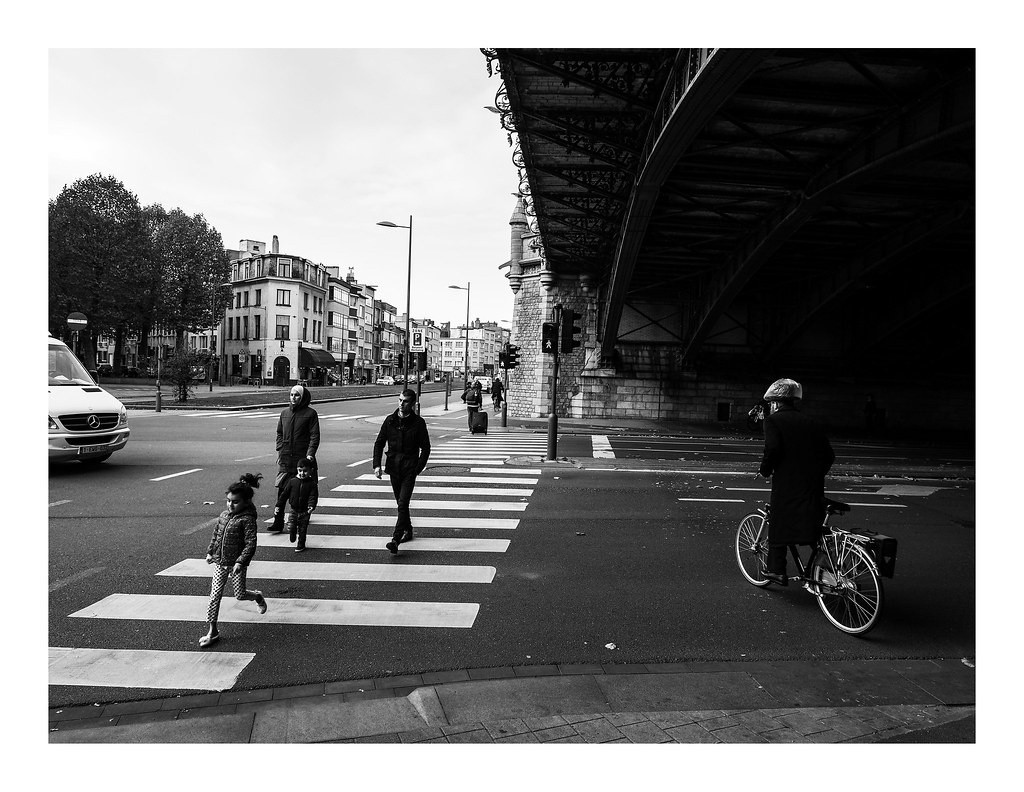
[48,330,132,466]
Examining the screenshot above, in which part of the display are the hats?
[763,378,802,401]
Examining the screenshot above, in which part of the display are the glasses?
[768,402,772,407]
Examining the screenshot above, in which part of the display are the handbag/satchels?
[850,528,897,579]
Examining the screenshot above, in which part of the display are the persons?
[265,385,321,531]
[198,472,268,645]
[461,379,482,431]
[273,458,318,552]
[490,378,504,408]
[760,378,834,588]
[373,389,431,556]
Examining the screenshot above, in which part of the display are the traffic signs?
[410,327,426,353]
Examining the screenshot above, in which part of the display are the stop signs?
[66,311,89,331]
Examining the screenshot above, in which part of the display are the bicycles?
[493,394,502,413]
[733,468,886,638]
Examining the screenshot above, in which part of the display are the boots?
[266,516,285,532]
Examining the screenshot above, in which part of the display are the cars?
[471,377,493,394]
[376,373,427,386]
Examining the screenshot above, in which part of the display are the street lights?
[448,281,471,403]
[208,282,233,392]
[375,214,414,392]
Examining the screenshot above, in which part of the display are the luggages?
[471,412,488,435]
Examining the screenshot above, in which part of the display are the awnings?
[301,348,337,368]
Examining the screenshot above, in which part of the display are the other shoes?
[290,532,297,542]
[254,590,267,614]
[199,630,221,646]
[400,528,413,543]
[386,542,398,553]
[295,542,306,552]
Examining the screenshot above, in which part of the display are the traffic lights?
[160,345,175,360]
[542,322,560,354]
[498,352,507,369]
[506,343,521,369]
[560,308,582,354]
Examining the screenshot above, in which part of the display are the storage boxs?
[851,527,897,577]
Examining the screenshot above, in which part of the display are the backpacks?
[466,388,477,403]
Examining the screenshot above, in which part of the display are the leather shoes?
[760,570,789,586]
